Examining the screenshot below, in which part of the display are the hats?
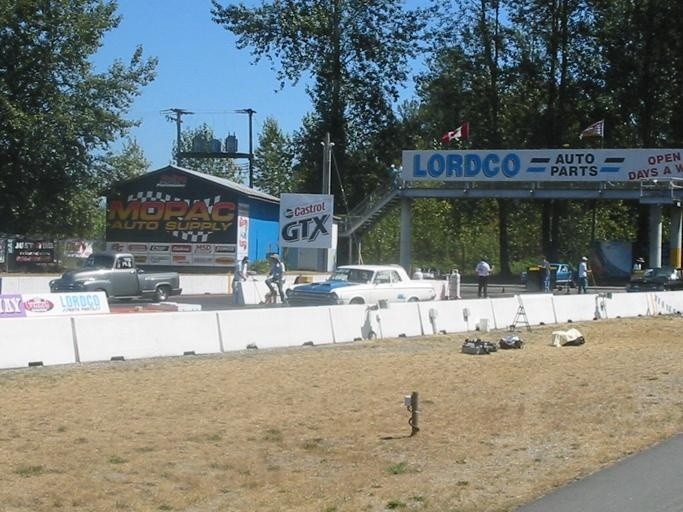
[270,253,279,262]
[636,257,645,263]
[582,256,588,261]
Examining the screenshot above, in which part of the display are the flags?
[578,119,603,145]
[441,121,469,144]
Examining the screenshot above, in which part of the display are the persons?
[539,257,550,292]
[230,256,250,306]
[262,253,288,308]
[577,256,594,294]
[632,257,644,270]
[474,257,495,299]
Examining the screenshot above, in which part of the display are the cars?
[624,265,681,291]
[282,262,435,305]
[519,262,575,284]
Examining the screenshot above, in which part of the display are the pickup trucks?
[50,252,181,304]
